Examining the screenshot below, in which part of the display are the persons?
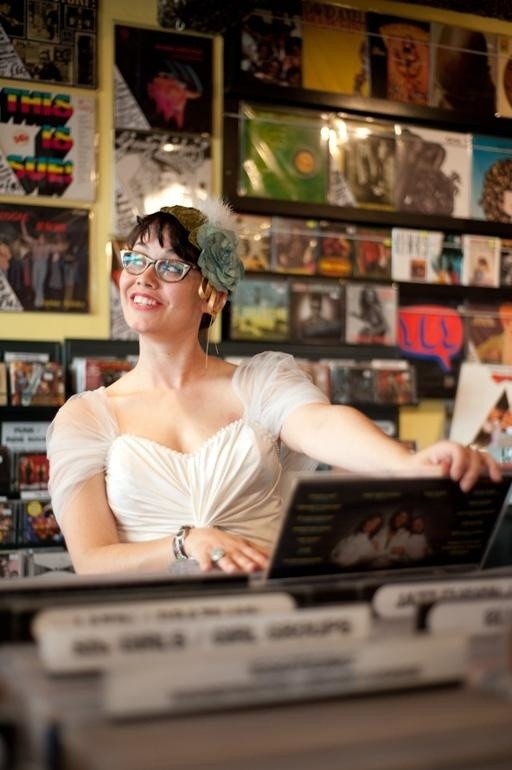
[350,287,389,344]
[300,292,330,329]
[329,508,430,569]
[20,219,70,311]
[42,204,512,577]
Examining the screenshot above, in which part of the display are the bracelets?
[172,524,196,561]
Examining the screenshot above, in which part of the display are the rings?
[210,547,226,562]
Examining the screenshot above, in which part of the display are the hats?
[161,203,245,293]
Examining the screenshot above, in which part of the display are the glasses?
[119,248,201,284]
